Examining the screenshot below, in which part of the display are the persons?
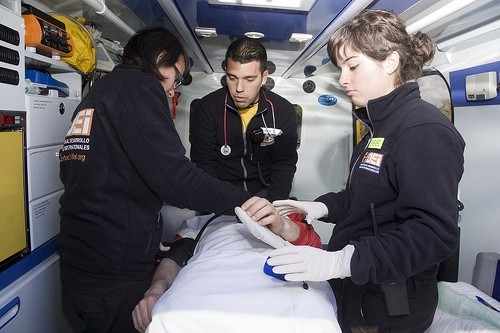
[267,9,466,333]
[56,26,253,333]
[189,36,299,202]
[131,196,341,333]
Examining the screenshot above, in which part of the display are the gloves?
[273,199,329,225]
[268,243,352,282]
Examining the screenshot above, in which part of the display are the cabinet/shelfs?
[0,0,125,333]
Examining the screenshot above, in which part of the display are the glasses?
[167,55,185,90]
